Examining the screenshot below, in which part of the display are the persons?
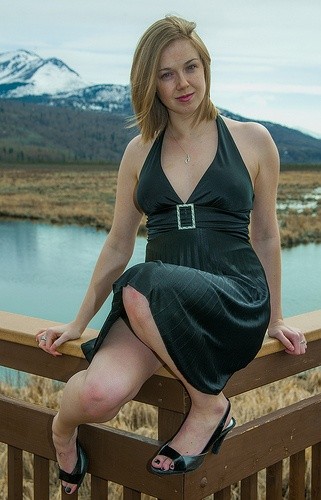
[35,15,308,495]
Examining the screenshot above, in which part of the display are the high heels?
[59,426,88,495]
[149,397,236,474]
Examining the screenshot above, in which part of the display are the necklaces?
[176,139,190,163]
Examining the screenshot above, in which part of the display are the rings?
[300,341,305,344]
[41,336,46,341]
